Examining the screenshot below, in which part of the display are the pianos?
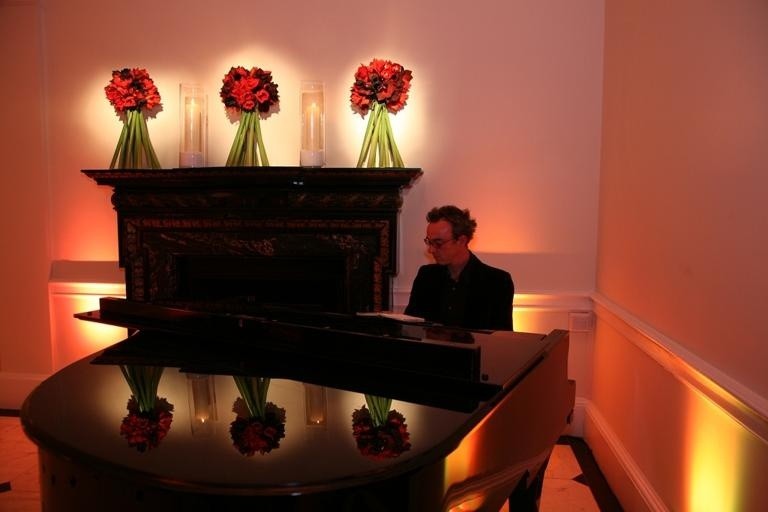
[21,297,576,512]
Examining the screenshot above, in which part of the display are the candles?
[192,380,209,422]
[185,96,200,152]
[306,384,323,423]
[308,106,319,151]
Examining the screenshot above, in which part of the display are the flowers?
[105,69,163,169]
[118,364,174,453]
[348,59,413,167]
[352,393,412,461]
[219,67,280,167]
[229,375,286,456]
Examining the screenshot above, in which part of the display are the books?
[356,311,426,323]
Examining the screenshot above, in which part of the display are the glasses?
[423,238,454,248]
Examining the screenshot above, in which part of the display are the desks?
[78,170,423,340]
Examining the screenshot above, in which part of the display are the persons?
[403,203,514,330]
